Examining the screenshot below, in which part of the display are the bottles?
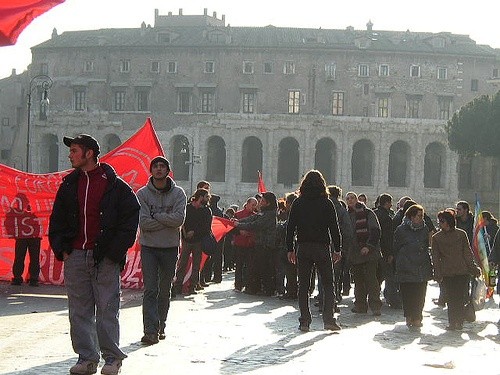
[488,267,496,287]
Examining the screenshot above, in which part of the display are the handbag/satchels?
[472,274,486,311]
[467,279,476,323]
[264,211,285,250]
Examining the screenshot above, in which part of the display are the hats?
[62,133,101,155]
[261,191,276,201]
[149,156,171,173]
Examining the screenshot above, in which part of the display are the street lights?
[180,133,194,195]
[26,75,53,172]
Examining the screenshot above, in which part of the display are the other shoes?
[280,293,298,300]
[434,301,445,308]
[300,323,308,331]
[233,269,235,270]
[141,335,159,346]
[243,291,248,294]
[177,277,214,299]
[445,323,463,330]
[229,270,232,271]
[406,319,422,328]
[159,334,165,339]
[11,277,23,285]
[323,322,340,331]
[27,279,39,287]
[351,306,367,313]
[101,360,122,375]
[373,312,381,316]
[70,359,97,375]
[234,289,240,292]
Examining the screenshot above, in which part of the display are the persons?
[392,205,433,327]
[49,133,140,375]
[11,239,40,287]
[172,190,212,296]
[286,170,343,331]
[190,182,500,316]
[431,210,481,330]
[135,156,187,344]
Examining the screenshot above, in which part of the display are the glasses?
[456,208,461,210]
